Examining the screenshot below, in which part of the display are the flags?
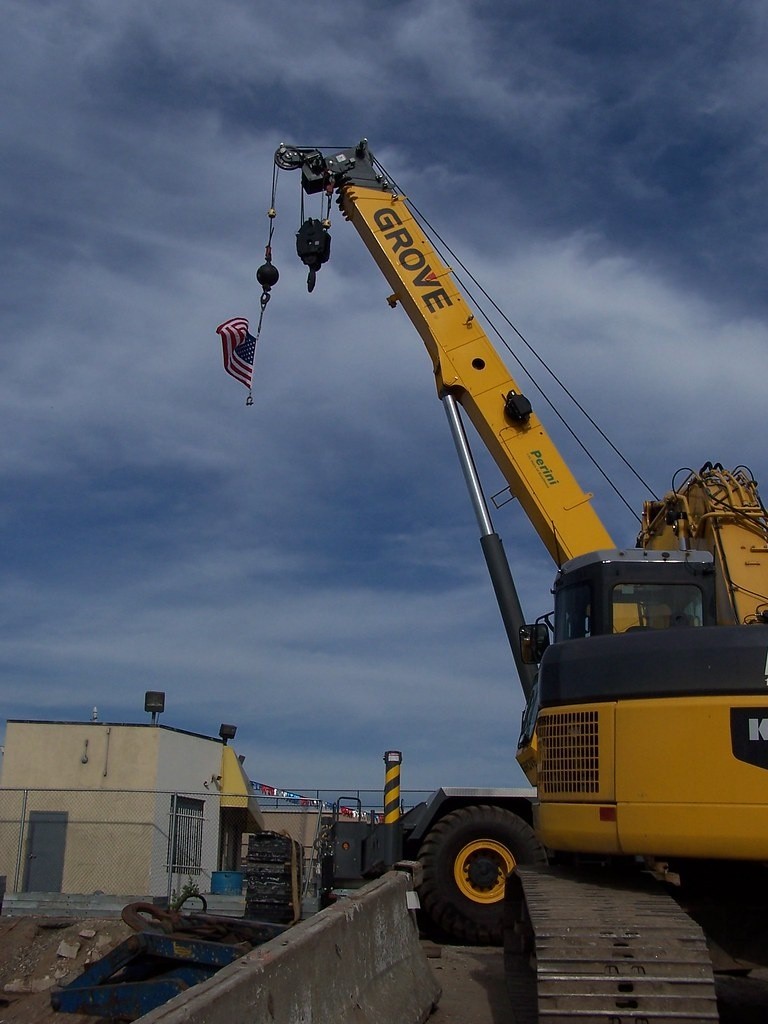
[217,319,255,392]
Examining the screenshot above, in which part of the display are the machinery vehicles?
[214,137,768,1024]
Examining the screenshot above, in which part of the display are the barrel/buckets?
[210,869,245,896]
